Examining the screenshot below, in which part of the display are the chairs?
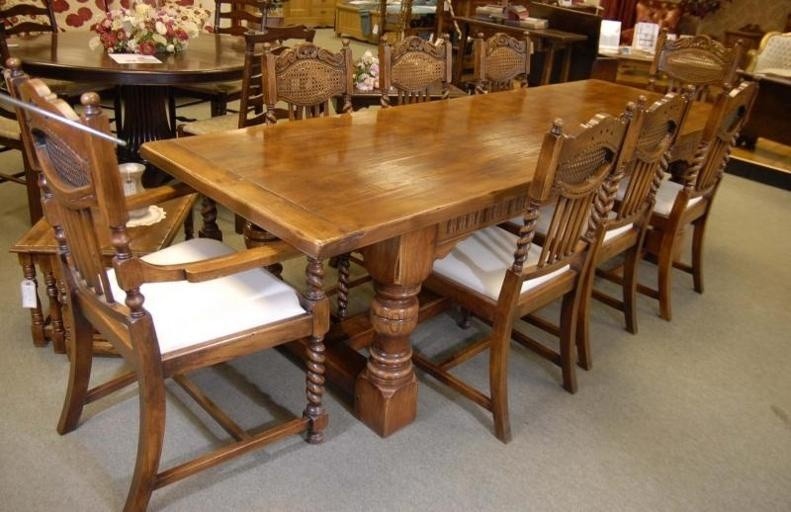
[0,2,532,357]
[5,55,331,511]
[645,81,761,294]
[529,84,697,372]
[413,101,637,443]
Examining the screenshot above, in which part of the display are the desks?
[453,13,589,88]
